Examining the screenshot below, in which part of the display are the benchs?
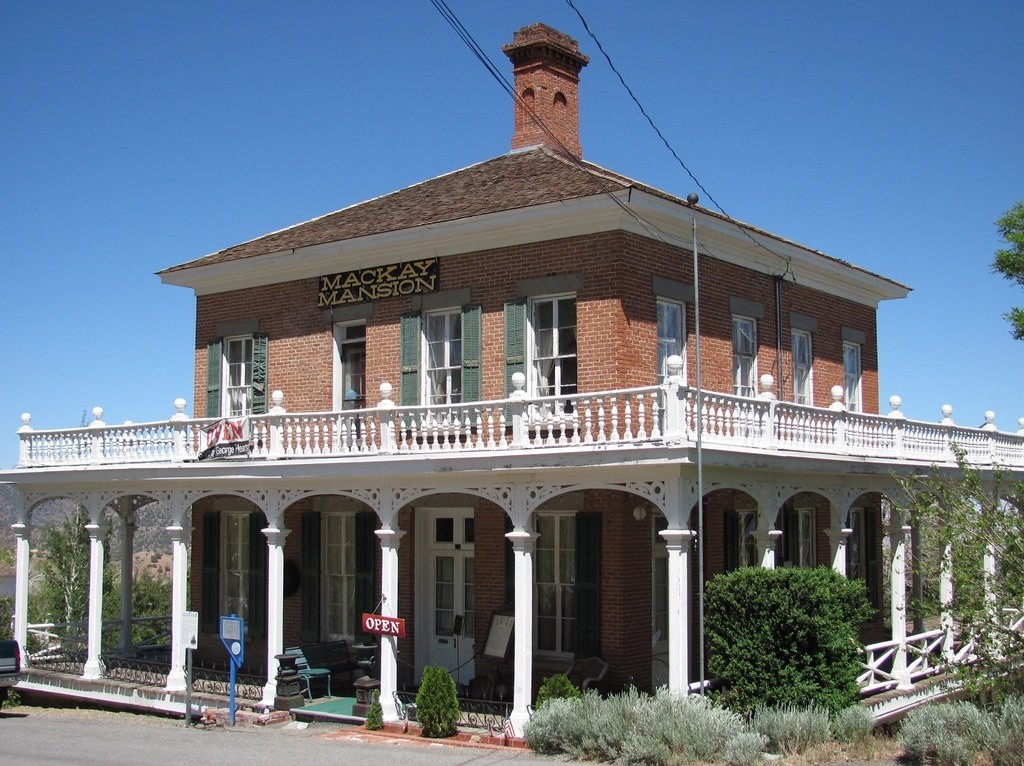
[284,639,362,699]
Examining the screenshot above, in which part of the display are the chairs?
[563,657,607,693]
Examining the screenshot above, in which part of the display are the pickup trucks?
[0,638,21,711]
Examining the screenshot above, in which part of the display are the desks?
[353,642,378,659]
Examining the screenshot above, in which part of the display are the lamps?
[633,505,647,522]
